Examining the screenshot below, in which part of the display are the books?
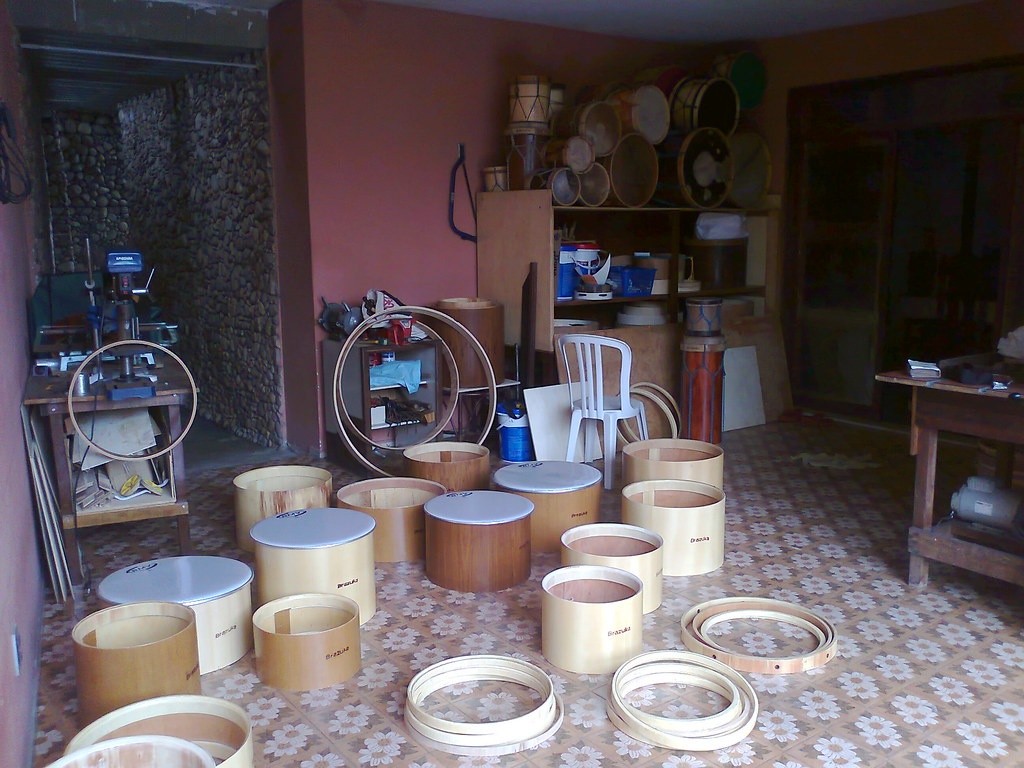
[907,359,941,378]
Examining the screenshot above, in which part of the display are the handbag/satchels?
[361,288,428,346]
[369,360,421,394]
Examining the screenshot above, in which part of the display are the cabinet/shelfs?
[321,339,443,479]
[476,189,768,352]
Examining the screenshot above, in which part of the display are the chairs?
[557,333,648,489]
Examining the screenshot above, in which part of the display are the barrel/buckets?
[557,244,578,300]
[571,242,600,287]
[496,401,535,463]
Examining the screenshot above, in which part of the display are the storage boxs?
[609,265,658,298]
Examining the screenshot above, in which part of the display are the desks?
[874,354,1024,589]
[442,379,521,442]
[23,355,200,586]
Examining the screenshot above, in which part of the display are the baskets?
[596,266,657,299]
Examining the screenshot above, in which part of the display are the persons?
[902,228,938,353]
